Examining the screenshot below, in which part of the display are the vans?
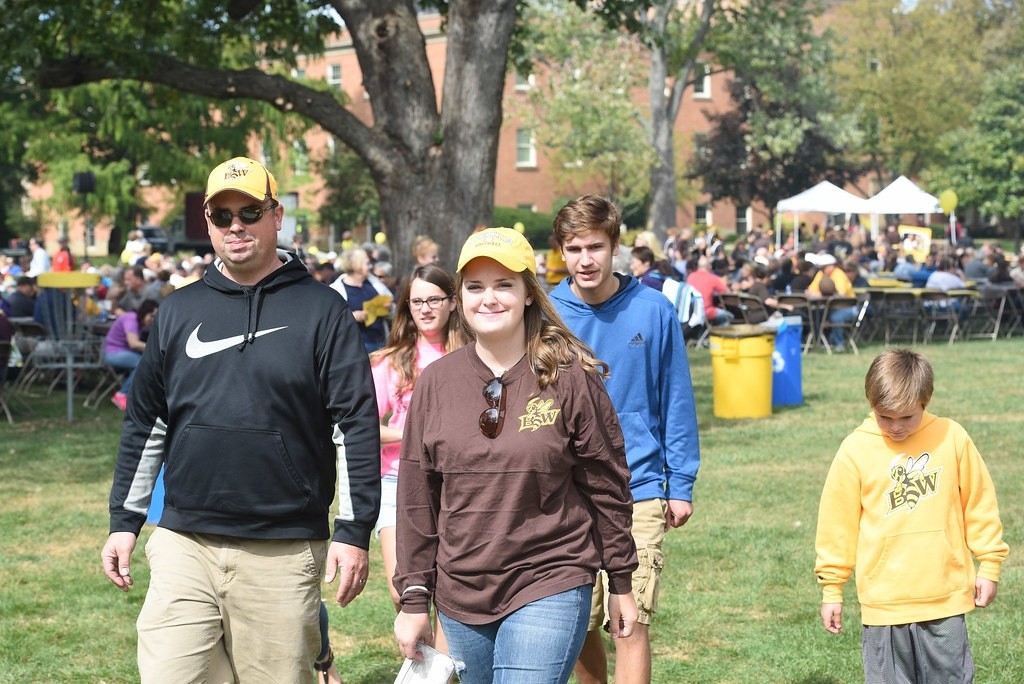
[109,227,168,255]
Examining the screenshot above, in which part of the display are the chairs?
[0,323,126,424]
[662,279,1024,356]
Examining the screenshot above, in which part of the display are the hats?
[17,277,37,286]
[203,156,279,208]
[326,252,337,260]
[456,227,535,277]
[814,254,836,265]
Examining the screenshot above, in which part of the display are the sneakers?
[111,391,126,410]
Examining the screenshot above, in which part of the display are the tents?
[868,175,960,248]
[775,180,867,260]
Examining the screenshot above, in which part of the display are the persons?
[815,350,1011,684]
[511,222,529,237]
[367,265,464,684]
[0,229,220,414]
[288,229,440,354]
[101,156,381,684]
[144,462,342,684]
[533,232,570,292]
[539,196,701,684]
[393,228,640,684]
[607,219,1024,352]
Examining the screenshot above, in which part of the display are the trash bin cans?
[708,324,779,419]
[758,314,804,406]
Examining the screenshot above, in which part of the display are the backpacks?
[649,272,705,339]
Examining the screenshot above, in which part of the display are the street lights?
[73,170,93,258]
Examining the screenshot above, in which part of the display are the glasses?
[478,376,503,439]
[406,295,451,310]
[207,203,276,228]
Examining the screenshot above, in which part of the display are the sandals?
[314,647,334,684]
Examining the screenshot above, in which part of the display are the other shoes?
[835,341,845,352]
